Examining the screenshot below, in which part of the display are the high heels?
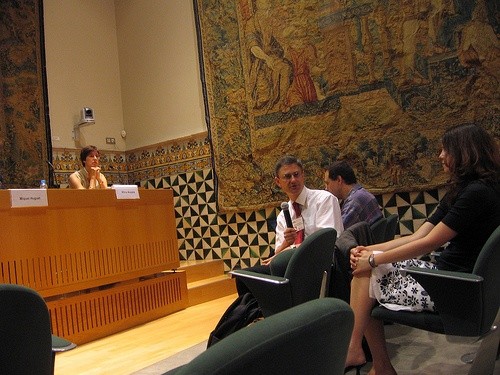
[343,362,366,375]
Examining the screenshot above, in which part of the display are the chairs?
[0,278,76,375]
[370,224,500,337]
[369,218,388,245]
[385,214,399,242]
[229,228,337,307]
[161,296,356,375]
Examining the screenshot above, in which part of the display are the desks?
[1,188,180,299]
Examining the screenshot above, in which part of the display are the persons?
[324,161,384,230]
[69,145,107,189]
[236,155,344,297]
[344,124,500,375]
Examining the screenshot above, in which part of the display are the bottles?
[40,179,49,190]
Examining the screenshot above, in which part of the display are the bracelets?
[368,255,373,268]
[290,245,296,249]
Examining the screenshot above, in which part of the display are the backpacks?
[206,292,262,349]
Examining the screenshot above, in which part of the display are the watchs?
[370,254,378,268]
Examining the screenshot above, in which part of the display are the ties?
[292,201,303,245]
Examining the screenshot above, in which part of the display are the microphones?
[109,164,126,184]
[46,159,58,188]
[281,202,293,228]
